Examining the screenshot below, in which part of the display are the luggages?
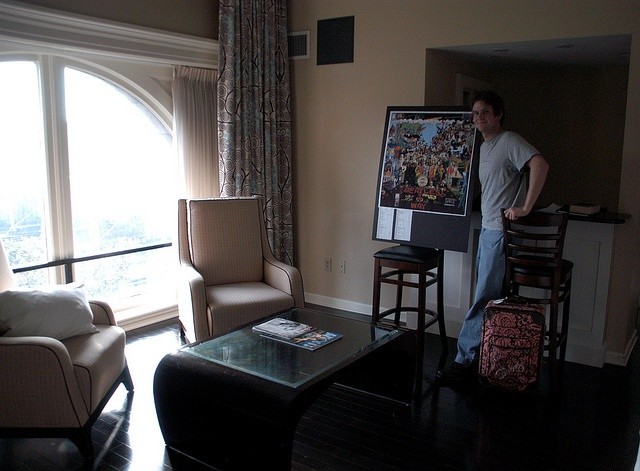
[478,296,545,391]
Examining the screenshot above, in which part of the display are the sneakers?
[437,361,468,380]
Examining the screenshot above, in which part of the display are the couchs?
[1,291,133,470]
[176,195,304,344]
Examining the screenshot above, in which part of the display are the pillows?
[1,291,100,340]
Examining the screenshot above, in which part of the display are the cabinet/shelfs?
[404,211,637,367]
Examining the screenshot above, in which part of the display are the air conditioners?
[288,30,311,60]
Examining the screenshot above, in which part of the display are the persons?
[437,90,549,381]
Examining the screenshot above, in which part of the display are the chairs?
[372,245,449,372]
[500,205,573,367]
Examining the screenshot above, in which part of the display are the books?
[260,322,343,351]
[556,205,589,218]
[252,316,317,340]
[570,202,601,215]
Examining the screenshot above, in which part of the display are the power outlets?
[337,260,346,274]
[324,258,332,271]
